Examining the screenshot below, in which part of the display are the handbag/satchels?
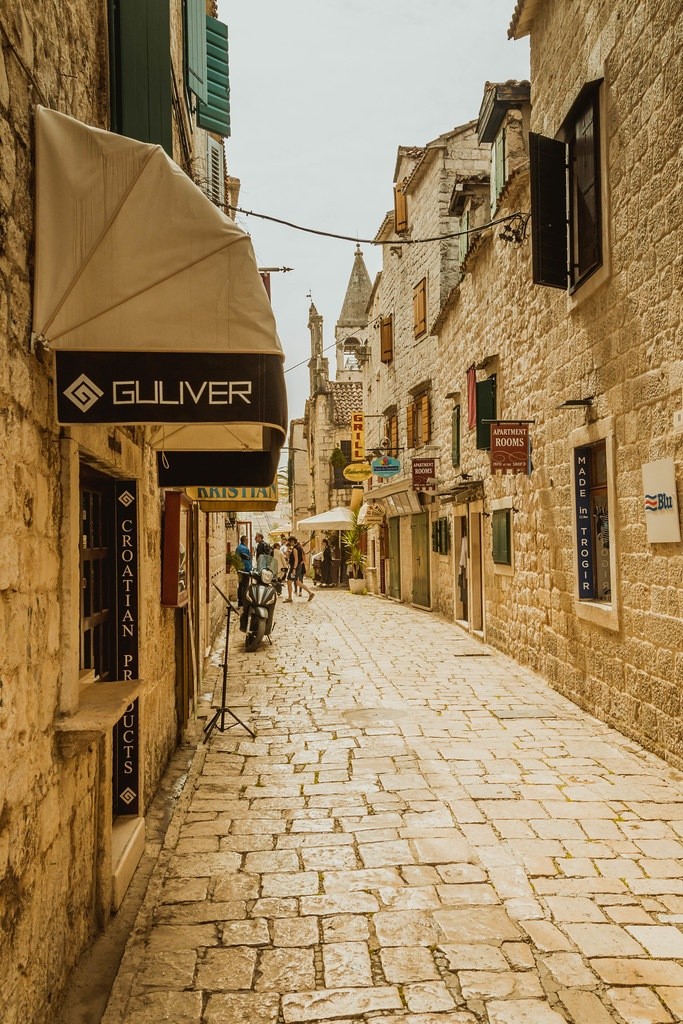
[302,562,306,573]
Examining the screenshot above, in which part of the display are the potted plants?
[339,504,369,595]
[330,447,347,485]
[226,551,244,582]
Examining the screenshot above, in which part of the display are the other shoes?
[238,601,244,606]
[293,591,297,595]
[308,593,315,601]
[299,592,302,595]
[282,599,292,603]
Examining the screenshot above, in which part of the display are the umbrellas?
[297,506,357,584]
[268,522,293,538]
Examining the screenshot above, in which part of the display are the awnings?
[184,468,278,512]
[31,105,289,487]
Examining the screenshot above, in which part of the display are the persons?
[235,535,253,608]
[321,539,335,588]
[273,536,315,603]
[255,532,271,562]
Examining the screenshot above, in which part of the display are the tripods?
[202,584,258,745]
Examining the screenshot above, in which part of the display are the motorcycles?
[235,554,291,653]
